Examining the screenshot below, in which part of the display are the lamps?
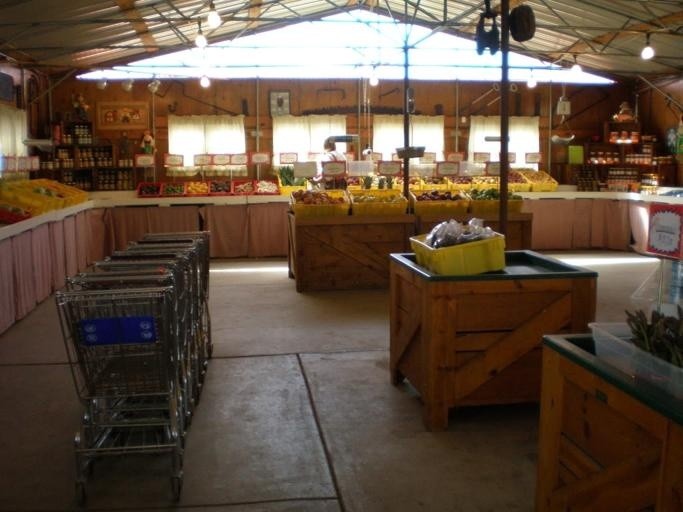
[195,1,224,48]
[96,69,161,95]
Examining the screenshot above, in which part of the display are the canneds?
[40,125,134,190]
[578,130,673,195]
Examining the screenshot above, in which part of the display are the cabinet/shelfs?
[284,207,537,289]
[535,332,683,512]
[1,119,154,329]
[389,247,597,430]
[111,121,674,258]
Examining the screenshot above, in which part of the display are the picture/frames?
[268,89,292,119]
[97,101,149,132]
